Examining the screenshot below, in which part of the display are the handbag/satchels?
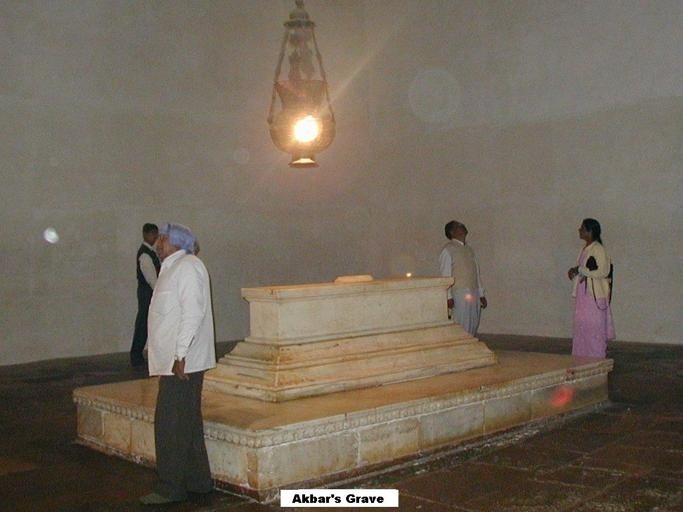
[586,256,613,280]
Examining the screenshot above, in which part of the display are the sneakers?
[139,491,186,505]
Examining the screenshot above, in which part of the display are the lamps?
[268,1,337,168]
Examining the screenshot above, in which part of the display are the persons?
[140,223,217,506]
[130,224,163,366]
[439,221,487,337]
[568,219,615,358]
